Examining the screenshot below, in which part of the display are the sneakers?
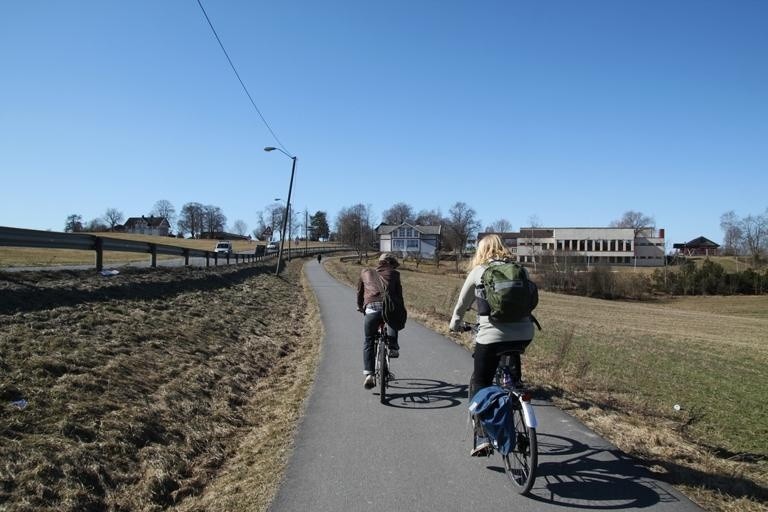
[387,349,400,359]
[467,438,493,457]
[363,375,377,390]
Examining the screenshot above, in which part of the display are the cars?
[255,241,280,256]
[215,240,232,253]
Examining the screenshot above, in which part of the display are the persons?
[317,254,321,263]
[356,252,408,389]
[448,232,535,456]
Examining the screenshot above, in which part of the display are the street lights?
[263,146,296,276]
[295,209,307,256]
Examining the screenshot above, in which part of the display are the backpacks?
[467,385,515,456]
[481,257,543,331]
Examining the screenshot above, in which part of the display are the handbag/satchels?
[380,282,408,331]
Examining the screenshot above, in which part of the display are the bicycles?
[449,313,538,495]
[355,305,401,403]
